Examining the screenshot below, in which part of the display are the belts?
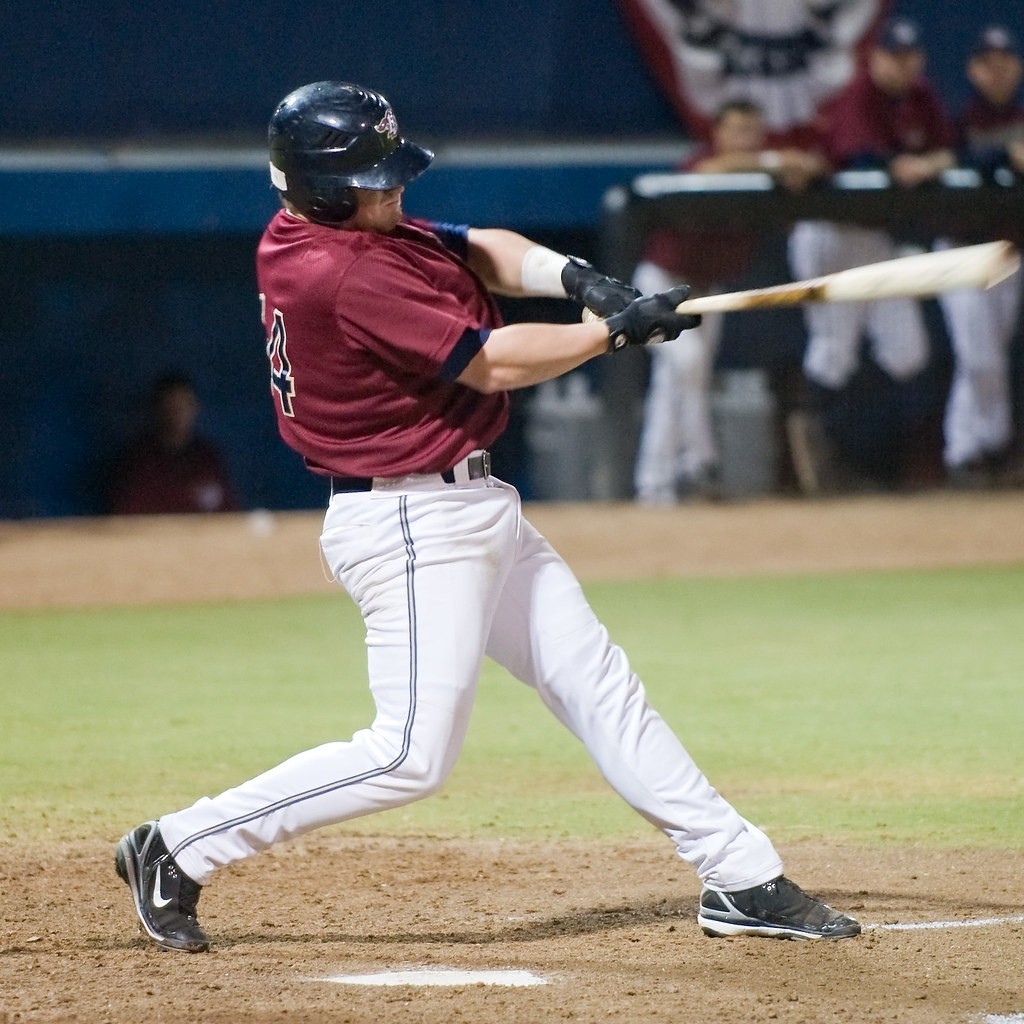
[333,450,492,494]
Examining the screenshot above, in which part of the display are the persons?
[631,22,1024,506]
[110,376,238,516]
[113,83,865,950]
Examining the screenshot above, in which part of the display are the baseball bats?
[581,239,1020,324]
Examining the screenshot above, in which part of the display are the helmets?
[873,14,925,52]
[965,22,1019,54]
[267,80,435,225]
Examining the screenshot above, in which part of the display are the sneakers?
[114,820,209,952]
[697,875,861,940]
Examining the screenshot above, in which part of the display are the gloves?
[604,285,704,355]
[561,254,644,318]
